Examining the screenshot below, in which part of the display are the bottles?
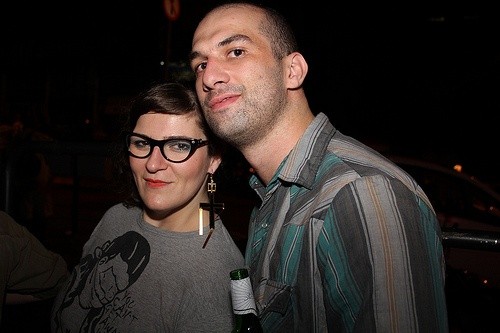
[230,269,263,333]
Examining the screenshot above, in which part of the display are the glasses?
[125,132,210,164]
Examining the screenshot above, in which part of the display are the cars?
[384,155,500,292]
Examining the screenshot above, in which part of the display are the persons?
[0,210,71,323]
[47,73,250,333]
[186,0,452,333]
